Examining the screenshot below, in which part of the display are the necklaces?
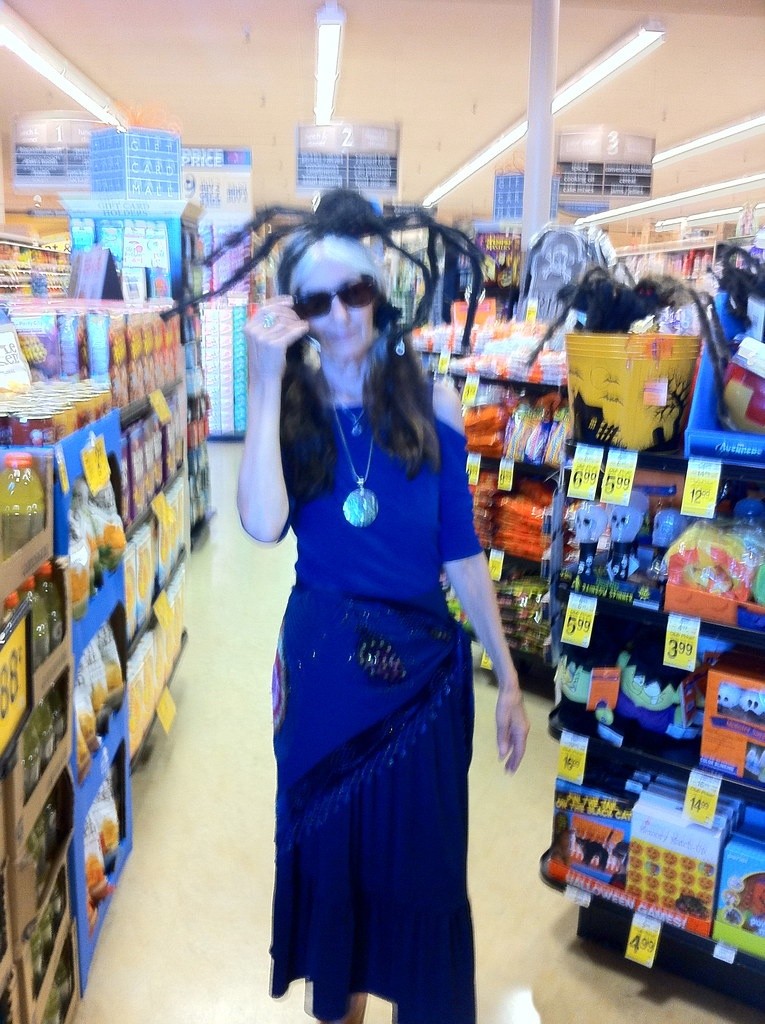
[316,349,387,529]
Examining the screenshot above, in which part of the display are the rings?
[263,316,275,328]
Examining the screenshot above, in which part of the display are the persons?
[235,235,531,1023]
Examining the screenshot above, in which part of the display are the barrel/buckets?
[565,329,704,449]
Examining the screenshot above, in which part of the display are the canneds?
[0,385,112,448]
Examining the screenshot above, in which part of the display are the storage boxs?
[625,665,765,944]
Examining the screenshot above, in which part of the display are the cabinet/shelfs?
[0,212,283,1024]
[540,246,765,1011]
[407,297,556,704]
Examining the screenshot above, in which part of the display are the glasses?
[293,281,375,319]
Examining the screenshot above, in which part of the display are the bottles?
[0,448,126,1024]
